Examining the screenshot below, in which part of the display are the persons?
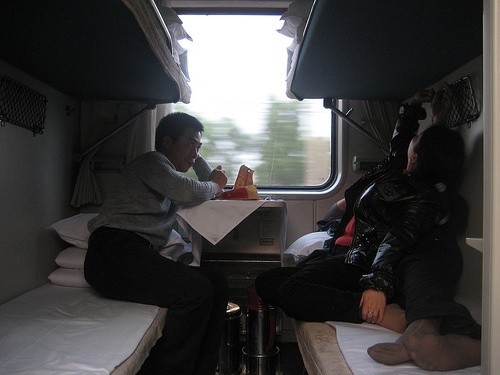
[255,86,468,340]
[82,111,231,375]
[323,196,482,371]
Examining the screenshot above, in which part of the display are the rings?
[367,311,373,313]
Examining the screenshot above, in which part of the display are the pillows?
[283,228,332,262]
[50,213,187,250]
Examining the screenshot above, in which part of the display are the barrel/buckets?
[213,302,241,373]
[242,298,280,357]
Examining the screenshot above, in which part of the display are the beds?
[275,0,483,375]
[0,0,193,375]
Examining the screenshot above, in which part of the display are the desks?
[192,201,286,208]
